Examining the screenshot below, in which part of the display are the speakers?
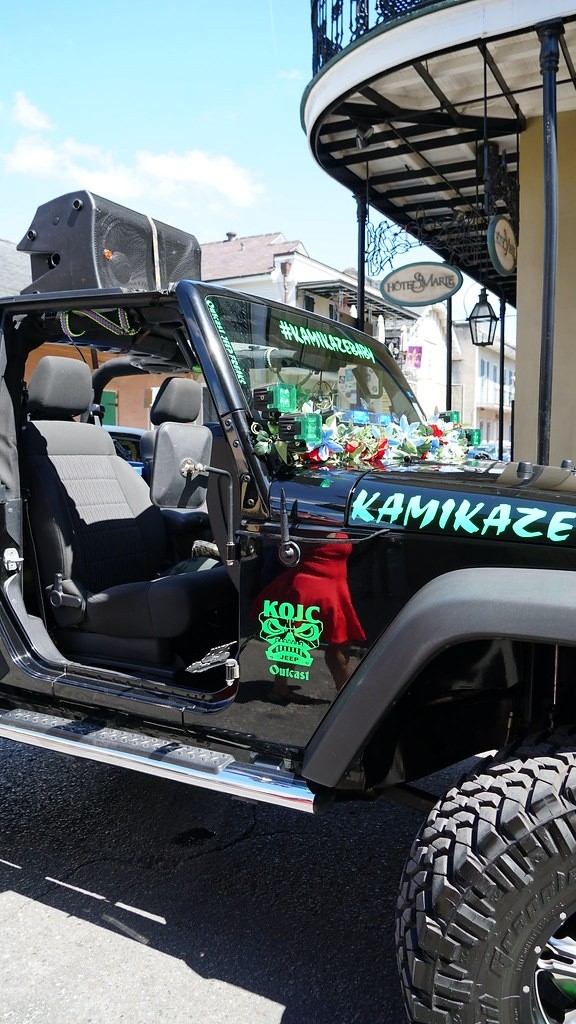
[16,189,202,295]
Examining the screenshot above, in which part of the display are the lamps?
[466,288,499,346]
[354,121,374,150]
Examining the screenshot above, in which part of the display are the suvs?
[0,279,576,1024]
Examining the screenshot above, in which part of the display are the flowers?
[252,415,472,471]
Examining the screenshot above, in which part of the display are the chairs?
[149,376,215,558]
[15,356,240,694]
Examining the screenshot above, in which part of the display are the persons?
[249,481,365,699]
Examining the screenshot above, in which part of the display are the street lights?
[465,287,507,461]
